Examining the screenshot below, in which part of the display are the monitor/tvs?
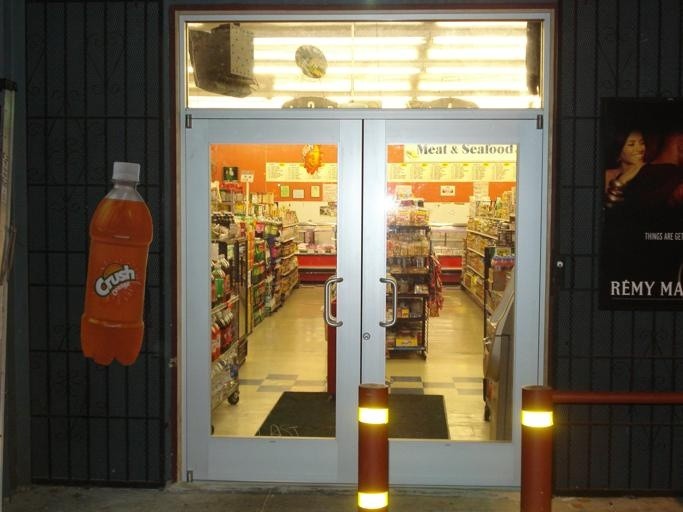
[189,25,255,96]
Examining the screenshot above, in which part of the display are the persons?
[599,119,650,210]
[606,122,683,297]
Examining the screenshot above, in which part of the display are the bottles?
[77,159,153,365]
[387,235,429,256]
[210,253,239,407]
[496,197,502,210]
[211,211,236,238]
[249,191,300,325]
[210,179,246,215]
[495,214,515,243]
[492,253,517,271]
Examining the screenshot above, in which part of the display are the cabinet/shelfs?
[460,214,516,316]
[243,202,299,334]
[386,225,431,360]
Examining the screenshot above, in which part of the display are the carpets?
[254,392,451,439]
